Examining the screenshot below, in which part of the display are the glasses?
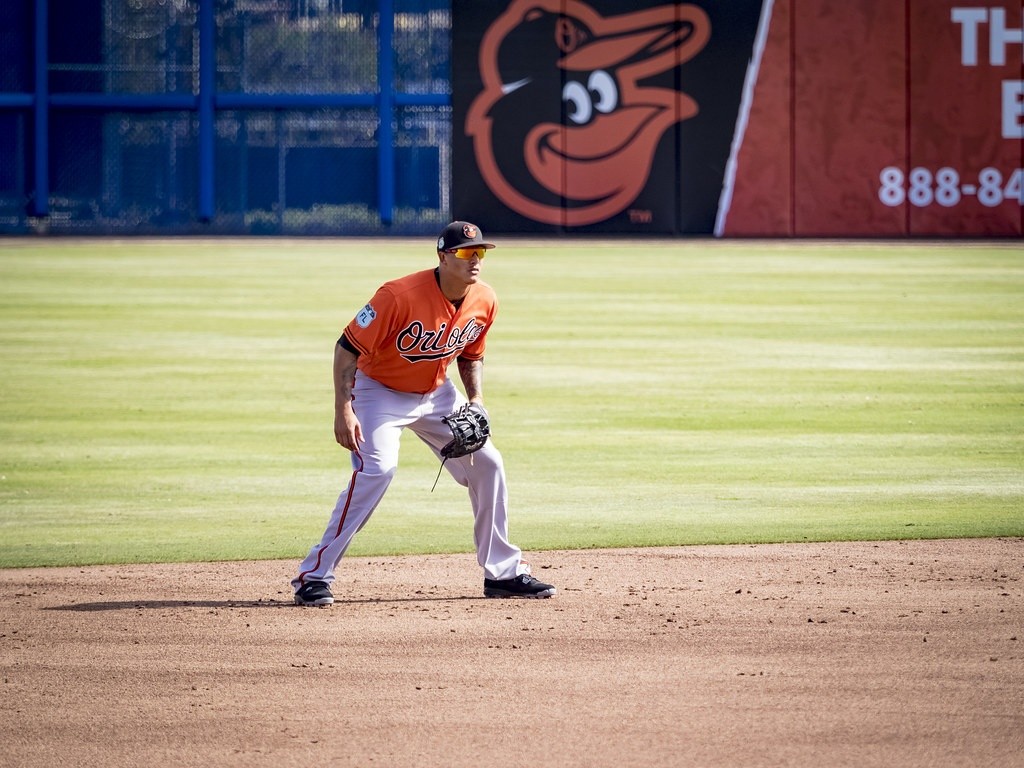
[444,245,486,259]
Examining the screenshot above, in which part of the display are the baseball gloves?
[440,402,490,459]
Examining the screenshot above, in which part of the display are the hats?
[437,220,496,252]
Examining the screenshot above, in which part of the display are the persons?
[291,221,557,607]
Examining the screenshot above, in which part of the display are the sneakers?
[293,581,334,607]
[483,573,556,599]
